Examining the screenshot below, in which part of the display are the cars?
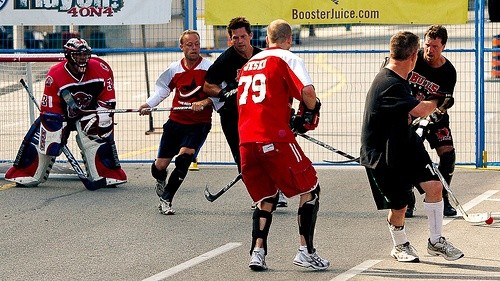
[249,25,303,46]
[1,26,106,49]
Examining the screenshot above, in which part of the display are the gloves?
[289,98,320,136]
[424,90,455,113]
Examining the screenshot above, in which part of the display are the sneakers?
[249,249,269,269]
[154,180,167,196]
[292,248,331,270]
[390,242,419,263]
[276,191,288,207]
[159,198,176,215]
[249,200,257,211]
[427,237,465,260]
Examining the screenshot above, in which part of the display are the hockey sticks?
[434,163,493,226]
[19,78,107,191]
[289,123,362,165]
[205,128,300,203]
[62,89,204,114]
[323,110,439,163]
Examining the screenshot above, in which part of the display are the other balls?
[485,217,493,225]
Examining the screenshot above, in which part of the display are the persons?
[402,25,457,219]
[5,36,128,190]
[359,31,465,263]
[203,17,289,209]
[138,30,228,215]
[234,20,330,272]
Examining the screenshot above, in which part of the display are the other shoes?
[443,201,456,216]
[406,200,415,218]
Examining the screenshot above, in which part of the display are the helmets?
[64,38,92,73]
